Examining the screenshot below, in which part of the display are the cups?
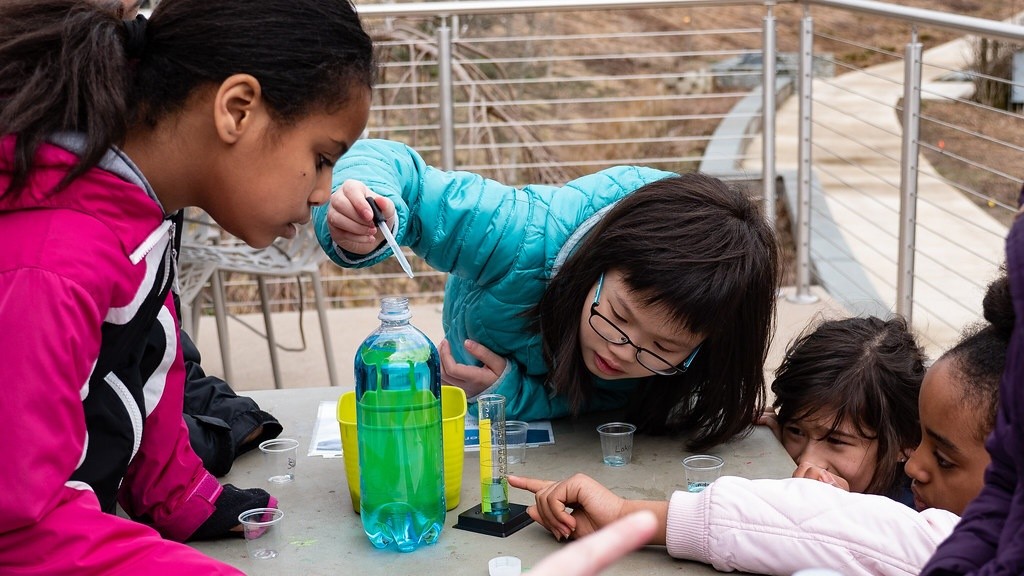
[258,438,299,485]
[682,454,724,493]
[596,422,636,467]
[238,508,285,560]
[491,420,529,465]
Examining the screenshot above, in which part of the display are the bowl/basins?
[335,386,467,515]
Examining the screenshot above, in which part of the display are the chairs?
[170,126,371,390]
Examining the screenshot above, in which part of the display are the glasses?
[588,271,700,377]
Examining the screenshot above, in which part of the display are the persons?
[918,183,1024,576]
[752,313,929,515]
[175,312,290,474]
[308,136,785,453]
[502,325,1024,576]
[0,0,378,575]
[515,510,660,576]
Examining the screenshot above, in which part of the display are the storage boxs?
[336,386,467,515]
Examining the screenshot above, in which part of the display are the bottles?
[353,296,446,553]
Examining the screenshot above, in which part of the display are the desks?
[118,386,799,576]
